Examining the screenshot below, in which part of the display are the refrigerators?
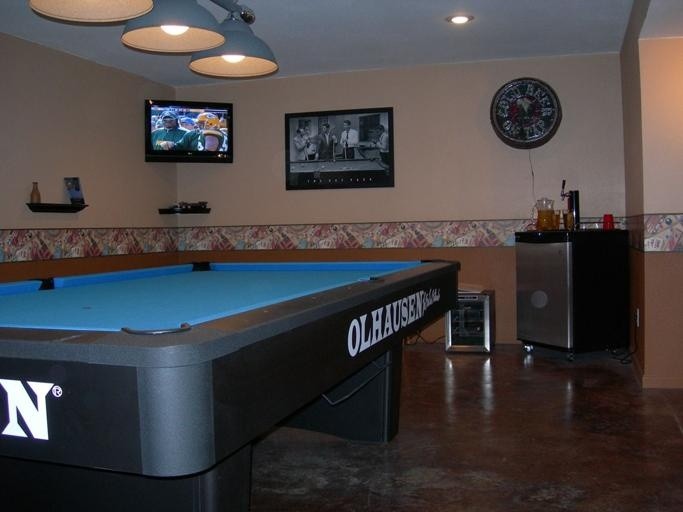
[514,228,632,354]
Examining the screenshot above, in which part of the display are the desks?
[2,258,463,512]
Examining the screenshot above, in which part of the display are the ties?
[346,131,348,146]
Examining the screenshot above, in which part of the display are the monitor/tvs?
[285,106,394,190]
[145,98,233,163]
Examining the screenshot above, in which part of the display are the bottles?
[30,181,41,203]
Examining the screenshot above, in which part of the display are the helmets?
[180,117,196,125]
[195,112,220,131]
[160,110,179,126]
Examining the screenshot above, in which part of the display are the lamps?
[26,1,279,81]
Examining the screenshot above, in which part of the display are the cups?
[561,208,576,232]
[554,210,560,230]
[602,214,615,232]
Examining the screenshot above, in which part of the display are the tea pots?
[528,197,557,233]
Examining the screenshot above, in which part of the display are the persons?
[339,119,357,159]
[361,123,388,165]
[151,108,228,153]
[313,122,337,161]
[305,125,316,160]
[293,127,308,160]
